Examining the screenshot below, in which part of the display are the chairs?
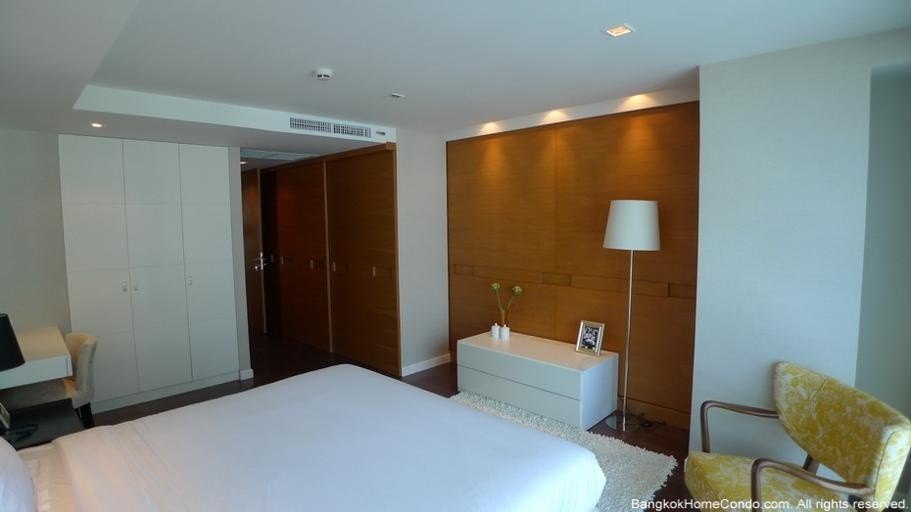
[683,362,910,511]
[0,333,97,430]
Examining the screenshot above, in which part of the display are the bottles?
[491,322,510,340]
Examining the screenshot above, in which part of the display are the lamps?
[0,313,24,372]
[601,199,661,433]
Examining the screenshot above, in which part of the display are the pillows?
[0,436,39,512]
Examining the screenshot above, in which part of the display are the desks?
[0,325,73,387]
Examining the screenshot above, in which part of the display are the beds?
[0,363,610,512]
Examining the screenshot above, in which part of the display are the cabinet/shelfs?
[456,330,619,431]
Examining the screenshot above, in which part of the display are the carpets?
[446,390,679,512]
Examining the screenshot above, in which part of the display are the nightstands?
[0,397,84,450]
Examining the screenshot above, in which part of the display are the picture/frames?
[576,320,606,357]
